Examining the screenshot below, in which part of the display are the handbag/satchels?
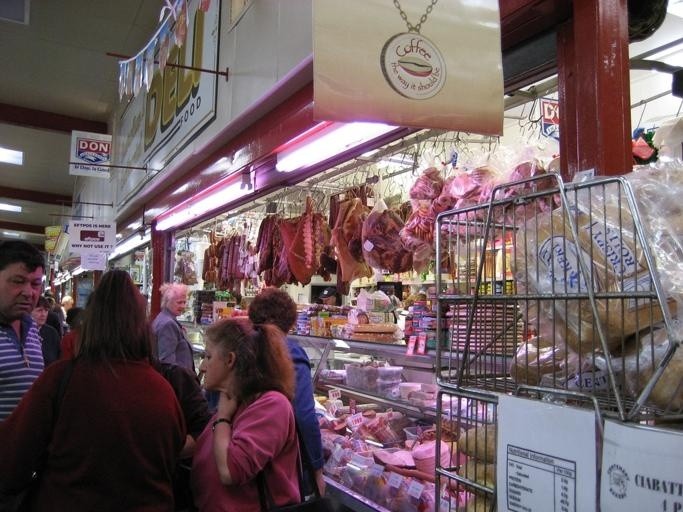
[258,496,335,512]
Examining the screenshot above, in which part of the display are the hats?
[318,287,338,299]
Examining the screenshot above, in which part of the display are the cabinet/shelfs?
[177,320,510,512]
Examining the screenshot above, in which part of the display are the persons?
[202,285,328,501]
[0,238,49,511]
[140,324,213,510]
[317,286,340,306]
[0,269,189,512]
[184,313,307,512]
[228,289,244,311]
[31,293,88,372]
[146,281,203,389]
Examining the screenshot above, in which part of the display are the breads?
[510,165,683,416]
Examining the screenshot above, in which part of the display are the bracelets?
[210,418,233,434]
